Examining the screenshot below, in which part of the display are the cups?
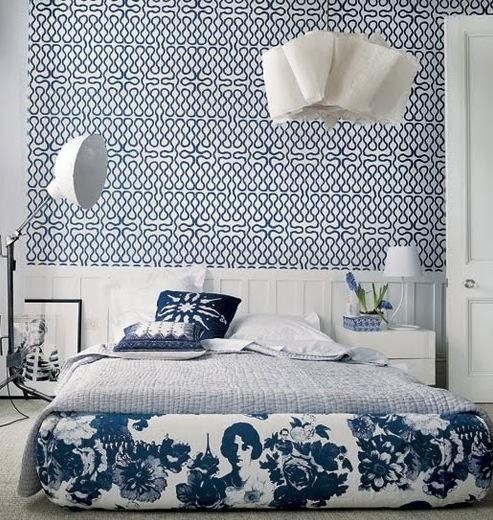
[346,302,360,314]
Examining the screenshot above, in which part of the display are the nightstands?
[335,324,437,386]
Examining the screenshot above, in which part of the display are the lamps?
[384,246,420,330]
[0,133,107,402]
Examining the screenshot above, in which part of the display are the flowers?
[345,271,394,324]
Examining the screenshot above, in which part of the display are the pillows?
[113,289,343,360]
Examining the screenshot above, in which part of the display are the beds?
[31,344,490,507]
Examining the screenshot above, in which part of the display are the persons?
[14,315,57,383]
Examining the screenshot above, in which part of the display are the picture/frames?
[24,299,81,398]
